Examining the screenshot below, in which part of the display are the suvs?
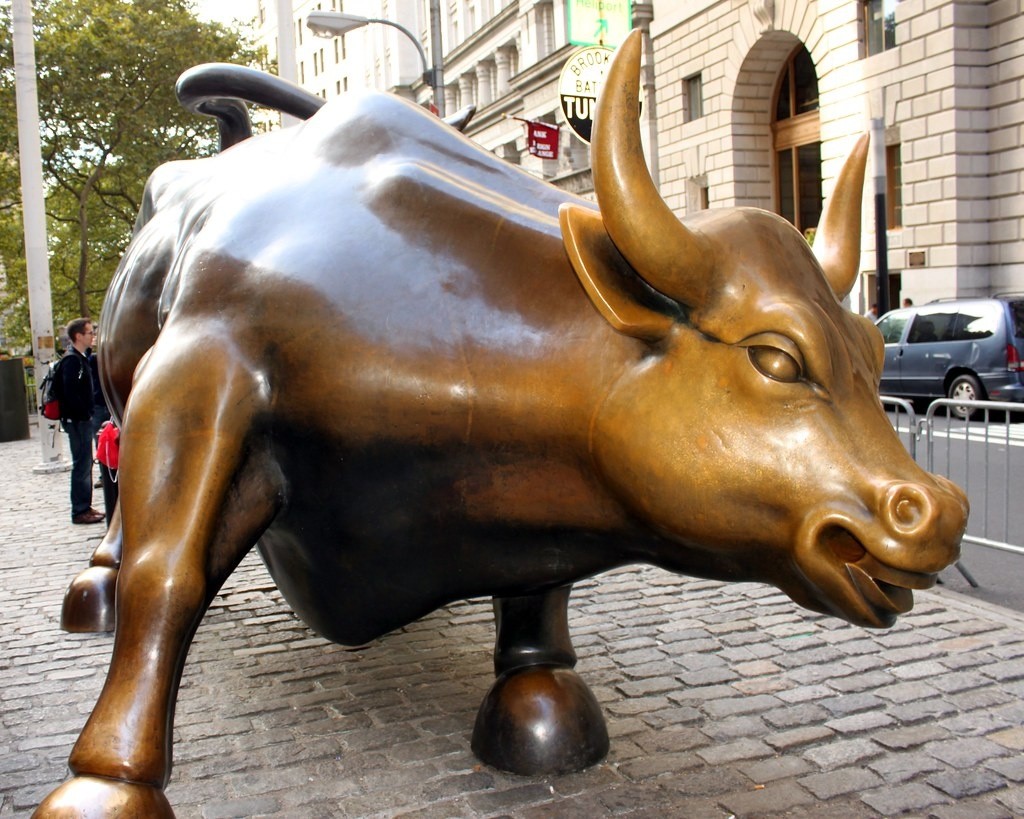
[867,291,1024,420]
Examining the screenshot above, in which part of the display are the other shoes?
[88,508,106,517]
[72,512,104,524]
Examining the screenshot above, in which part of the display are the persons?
[902,299,913,308]
[51,317,112,524]
[863,304,878,326]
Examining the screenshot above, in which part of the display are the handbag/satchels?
[95,415,120,482]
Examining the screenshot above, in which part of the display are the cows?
[28,29,970,819]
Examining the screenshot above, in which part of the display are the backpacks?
[38,352,96,421]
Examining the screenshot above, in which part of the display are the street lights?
[305,1,445,118]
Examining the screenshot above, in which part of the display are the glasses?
[84,331,97,336]
